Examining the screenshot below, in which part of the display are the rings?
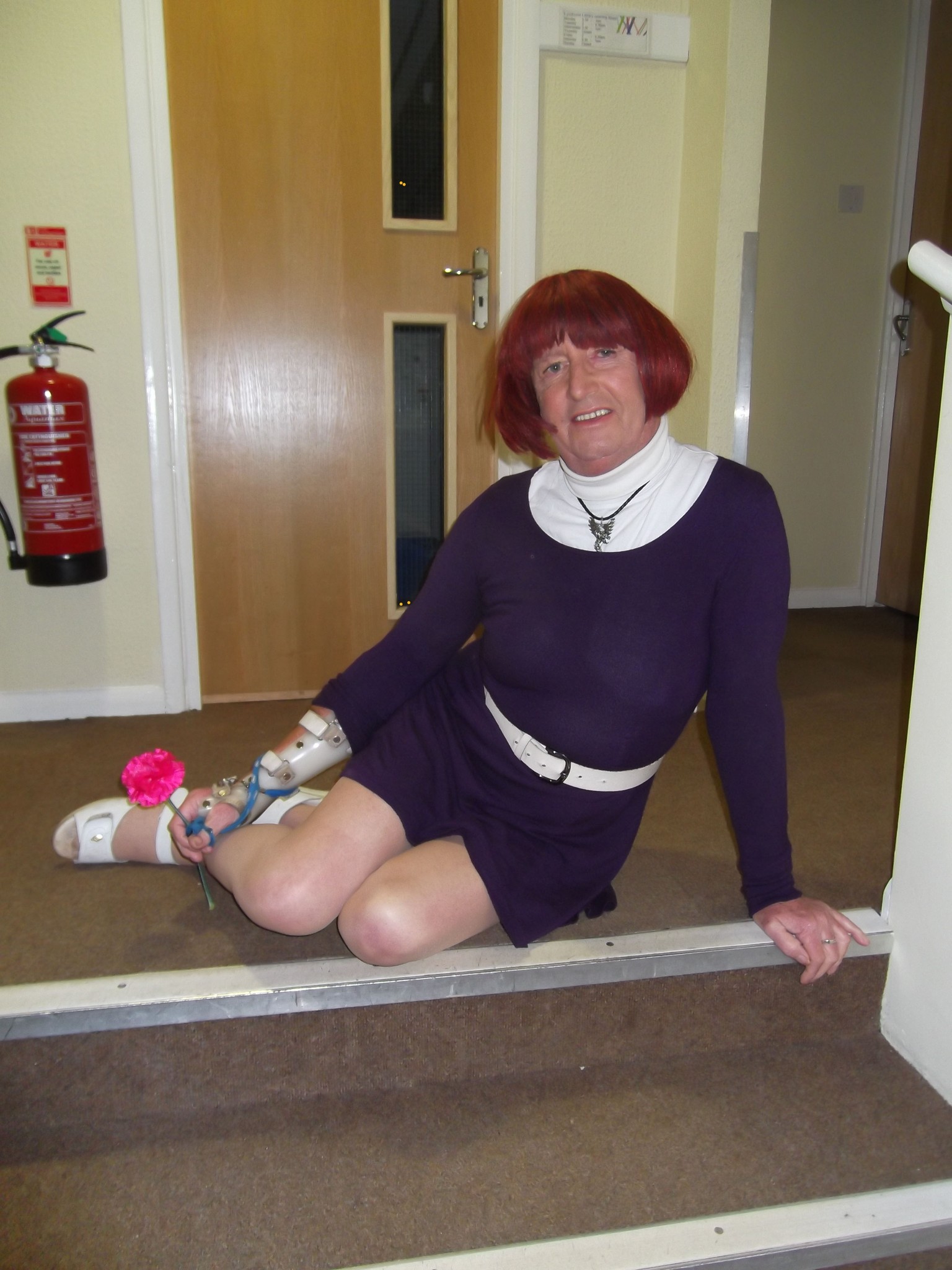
[823,938,836,945]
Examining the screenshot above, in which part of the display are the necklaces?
[576,481,653,552]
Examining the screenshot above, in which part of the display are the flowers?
[124,748,216,915]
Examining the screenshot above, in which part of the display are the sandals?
[53,788,189,864]
[250,786,330,824]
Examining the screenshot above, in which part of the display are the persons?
[53,268,867,990]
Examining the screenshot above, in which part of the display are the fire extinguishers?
[0,312,113,587]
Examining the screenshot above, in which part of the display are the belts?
[486,691,670,792]
